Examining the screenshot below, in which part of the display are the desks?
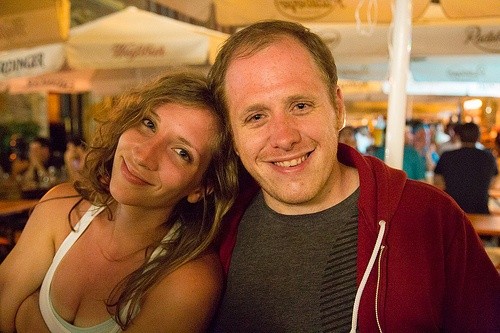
[0,198,38,217]
[467,214,499,237]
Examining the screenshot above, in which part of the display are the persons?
[64,140,84,179]
[20,137,55,189]
[434,124,498,214]
[0,70,240,333]
[337,113,500,188]
[206,21,500,333]
